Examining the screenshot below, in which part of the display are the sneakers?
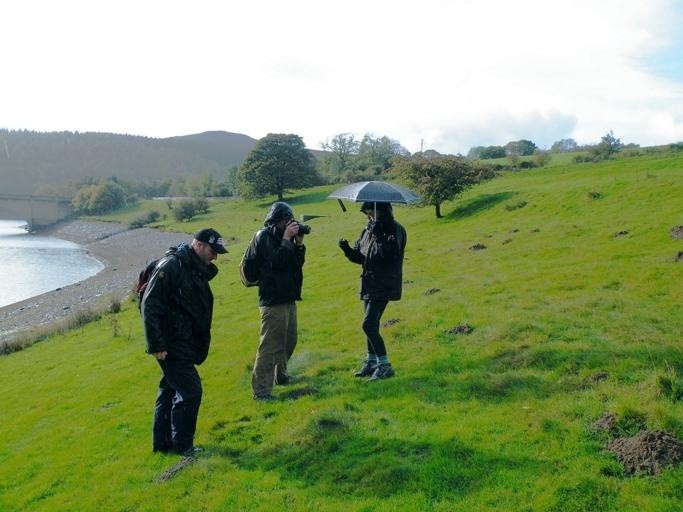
[276,375,299,385]
[354,359,379,378]
[253,394,277,401]
[367,362,396,383]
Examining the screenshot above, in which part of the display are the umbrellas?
[326,179,420,222]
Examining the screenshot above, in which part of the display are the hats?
[194,226,230,255]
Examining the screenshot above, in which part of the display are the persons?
[141,227,230,456]
[240,201,305,401]
[338,201,406,382]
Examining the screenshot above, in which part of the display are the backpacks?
[238,229,274,288]
[136,247,183,318]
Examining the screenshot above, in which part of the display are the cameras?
[290,219,310,237]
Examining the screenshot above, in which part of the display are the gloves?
[338,236,350,251]
[367,220,382,237]
